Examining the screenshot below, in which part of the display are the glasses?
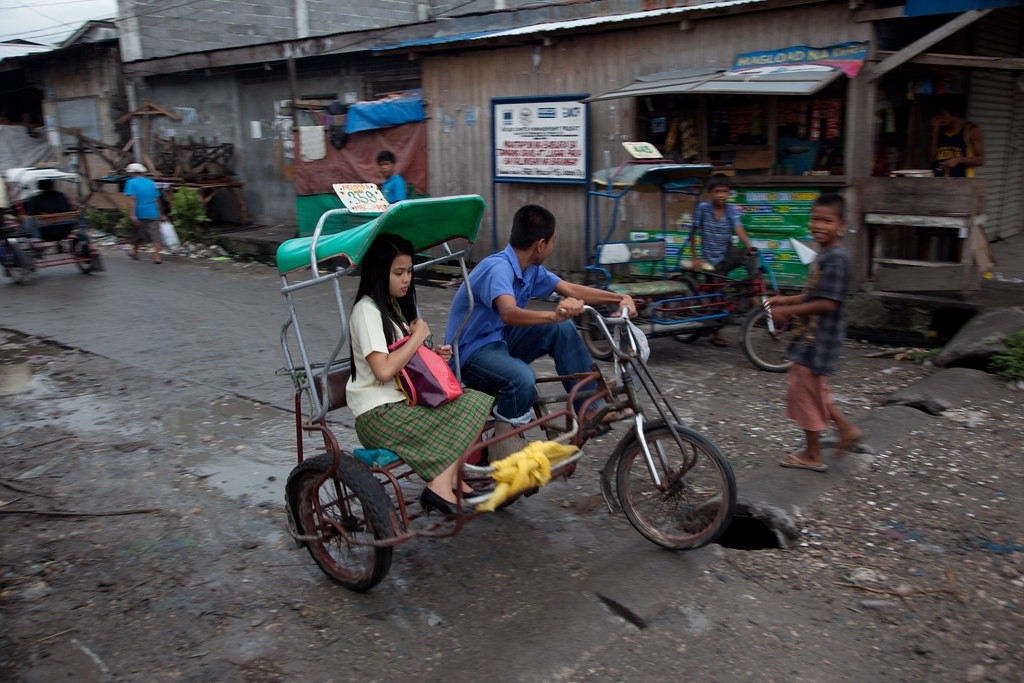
[423,334,434,349]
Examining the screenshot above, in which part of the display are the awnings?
[578,61,862,102]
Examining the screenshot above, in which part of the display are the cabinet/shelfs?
[698,95,777,176]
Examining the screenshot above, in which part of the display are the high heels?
[419,486,473,514]
[458,484,494,505]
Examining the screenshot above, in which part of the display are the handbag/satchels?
[387,335,463,408]
[159,218,182,248]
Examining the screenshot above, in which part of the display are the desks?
[94,176,245,238]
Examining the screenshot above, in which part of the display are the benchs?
[595,241,691,306]
[8,212,89,238]
[313,347,472,474]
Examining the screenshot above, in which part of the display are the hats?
[126,164,148,174]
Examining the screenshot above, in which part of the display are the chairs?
[154,134,234,182]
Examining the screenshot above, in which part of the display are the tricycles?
[272,192,739,594]
[578,160,813,375]
[0,166,108,287]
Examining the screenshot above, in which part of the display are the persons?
[691,172,761,347]
[928,94,995,279]
[443,206,635,429]
[345,234,495,520]
[22,177,74,241]
[762,192,864,472]
[377,150,406,204]
[124,163,169,264]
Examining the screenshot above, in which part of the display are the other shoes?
[127,250,139,260]
[153,256,163,264]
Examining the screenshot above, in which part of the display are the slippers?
[576,402,635,428]
[779,452,828,473]
